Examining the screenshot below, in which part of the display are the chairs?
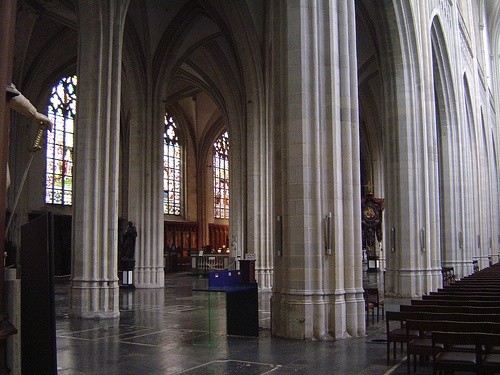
[368,288,384,322]
[363,289,374,326]
[386,262,500,375]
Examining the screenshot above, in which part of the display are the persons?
[123,221,137,259]
